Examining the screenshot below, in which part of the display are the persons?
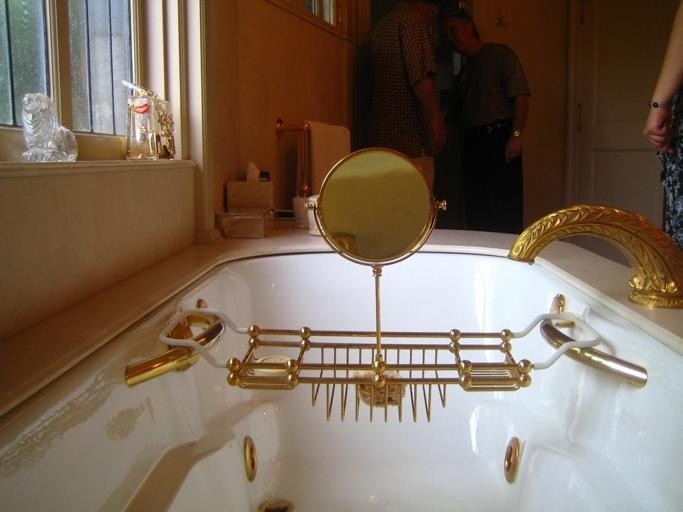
[437,7,530,233]
[359,1,448,194]
[641,0,682,256]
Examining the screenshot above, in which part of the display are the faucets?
[507,204,683,308]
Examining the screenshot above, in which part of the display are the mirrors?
[306,149,448,388]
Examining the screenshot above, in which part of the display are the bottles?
[152,99,176,160]
[125,96,158,162]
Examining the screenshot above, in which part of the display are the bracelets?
[646,99,673,111]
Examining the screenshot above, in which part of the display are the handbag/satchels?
[468,119,504,164]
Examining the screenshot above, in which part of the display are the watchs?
[510,128,523,138]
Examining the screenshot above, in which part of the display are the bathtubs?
[2,229,682,510]
